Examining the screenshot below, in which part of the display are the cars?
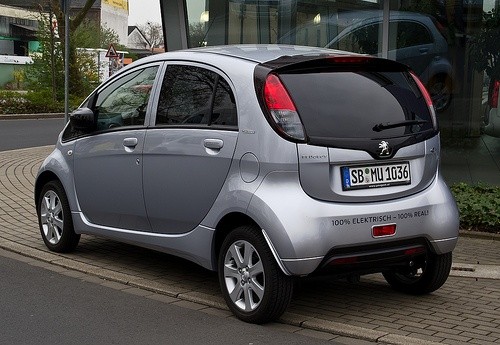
[34,43,461,324]
[269,7,454,115]
